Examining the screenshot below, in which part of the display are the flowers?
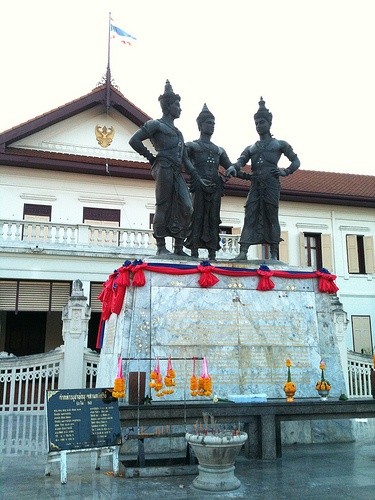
[189,355,213,397]
[148,354,176,400]
[112,354,127,399]
[283,358,296,392]
[315,359,331,391]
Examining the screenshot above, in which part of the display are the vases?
[318,390,328,401]
[285,392,295,403]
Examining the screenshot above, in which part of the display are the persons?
[183,103,252,261]
[224,96,300,262]
[128,79,217,260]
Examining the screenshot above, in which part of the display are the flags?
[109,18,139,49]
